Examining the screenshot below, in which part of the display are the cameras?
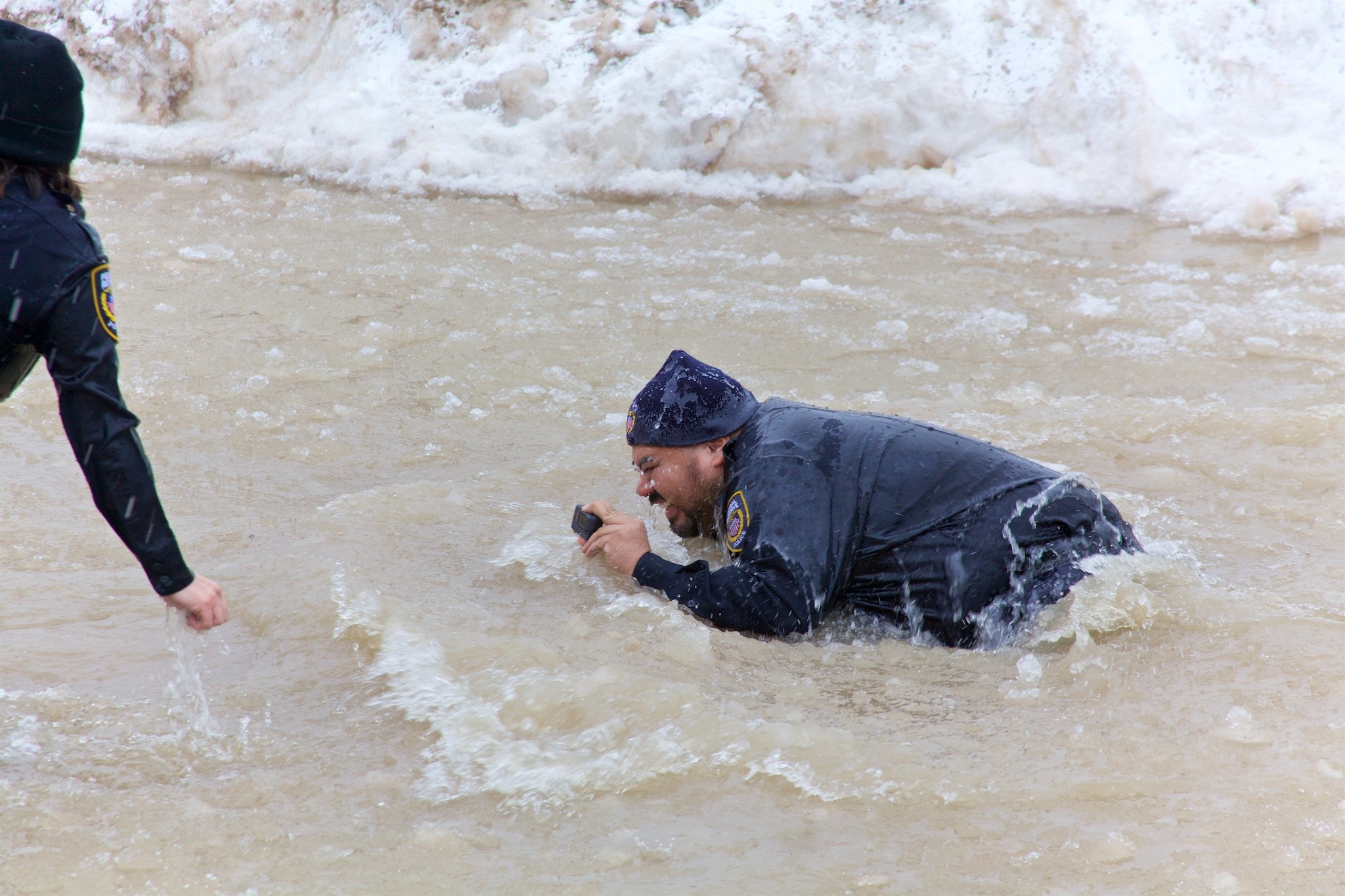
[571,504,603,540]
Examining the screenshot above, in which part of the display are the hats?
[626,351,760,446]
[0,19,84,176]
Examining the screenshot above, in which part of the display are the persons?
[578,351,1144,647]
[0,18,227,630]
